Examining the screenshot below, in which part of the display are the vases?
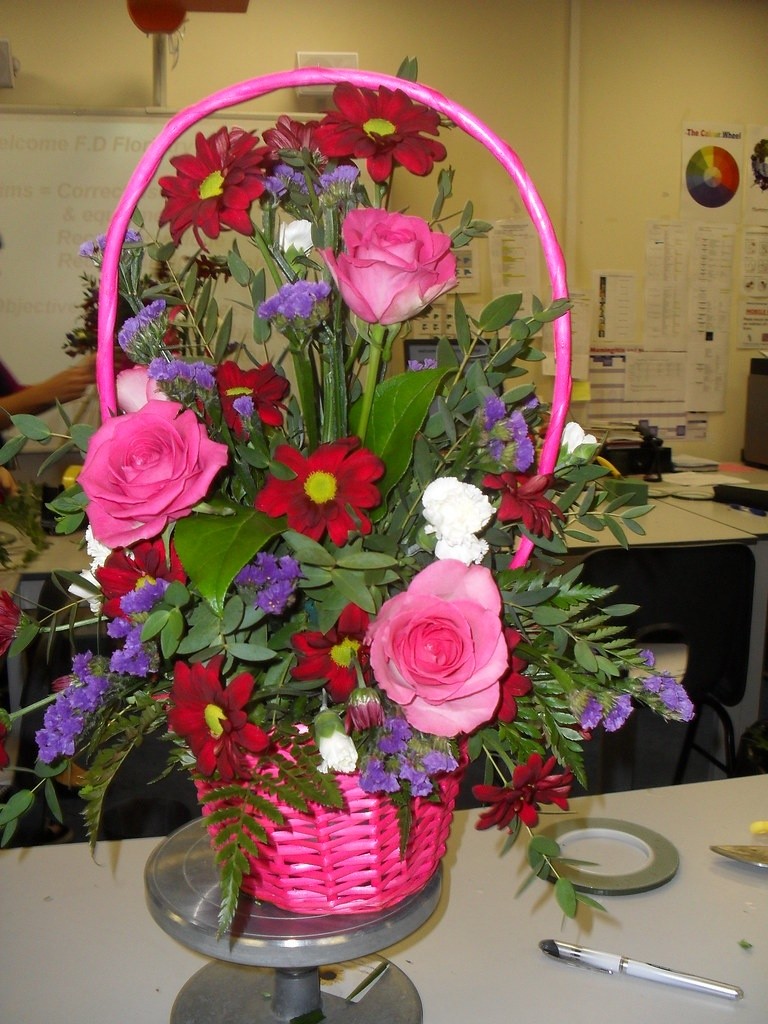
[192,715,474,916]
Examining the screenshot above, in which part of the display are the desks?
[0,773,766,1020]
[0,442,767,795]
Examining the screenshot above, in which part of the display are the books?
[589,421,643,449]
[672,454,719,472]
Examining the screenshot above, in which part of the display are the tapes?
[648,488,715,501]
[528,816,680,896]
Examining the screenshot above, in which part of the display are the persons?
[0,355,96,431]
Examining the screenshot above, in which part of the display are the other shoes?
[54,771,93,787]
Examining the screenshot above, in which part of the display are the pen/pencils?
[729,503,767,517]
[538,937,744,1002]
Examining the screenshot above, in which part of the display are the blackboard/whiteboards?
[0,103,387,454]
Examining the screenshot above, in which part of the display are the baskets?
[96,68,574,916]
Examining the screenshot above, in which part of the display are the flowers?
[0,68,697,936]
